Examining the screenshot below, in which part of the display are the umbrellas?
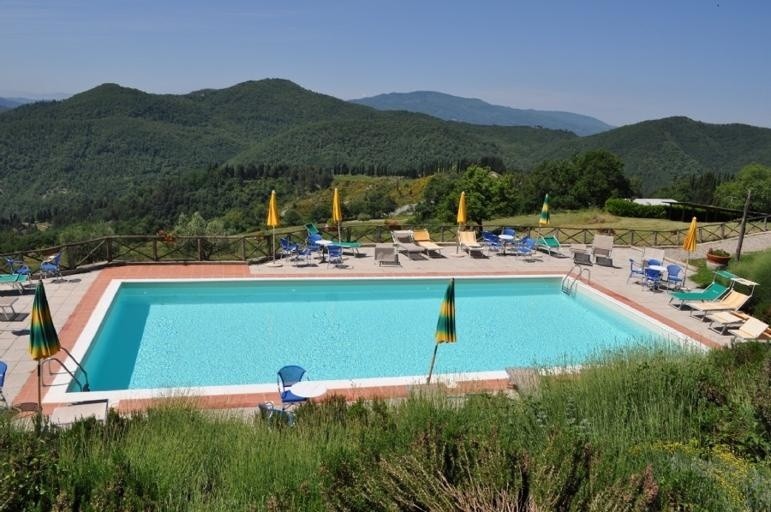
[330,186,345,245]
[538,191,556,241]
[267,187,285,264]
[425,276,460,382]
[454,191,471,254]
[28,281,63,426]
[679,215,701,287]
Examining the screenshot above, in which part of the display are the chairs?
[629,247,769,348]
[570,235,617,267]
[391,230,425,261]
[376,243,401,269]
[0,248,63,408]
[457,230,484,257]
[279,228,362,270]
[413,230,444,258]
[258,365,309,426]
[481,228,564,261]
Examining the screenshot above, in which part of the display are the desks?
[291,380,328,406]
